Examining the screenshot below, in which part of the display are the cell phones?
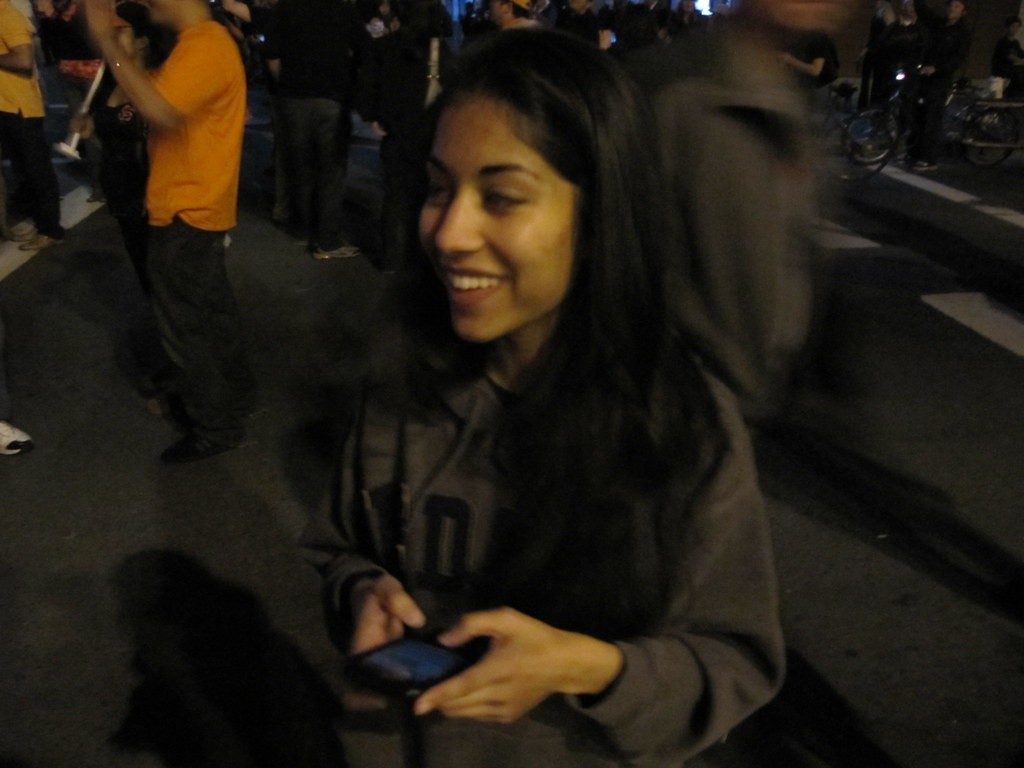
[344,624,493,701]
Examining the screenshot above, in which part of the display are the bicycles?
[812,59,1024,171]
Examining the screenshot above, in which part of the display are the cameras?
[610,32,617,43]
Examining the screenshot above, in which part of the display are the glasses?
[113,25,132,34]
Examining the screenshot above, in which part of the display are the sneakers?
[0,421,34,455]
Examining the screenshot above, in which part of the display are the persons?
[75,0,247,460]
[595,0,855,393]
[461,0,715,57]
[778,28,838,89]
[296,28,788,768]
[0,0,458,261]
[857,0,1024,170]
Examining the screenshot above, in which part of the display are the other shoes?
[12,226,38,241]
[19,235,65,250]
[915,161,940,171]
[892,152,912,165]
[162,428,247,463]
[312,244,362,259]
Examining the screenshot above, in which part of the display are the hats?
[512,0,532,9]
[115,3,151,39]
